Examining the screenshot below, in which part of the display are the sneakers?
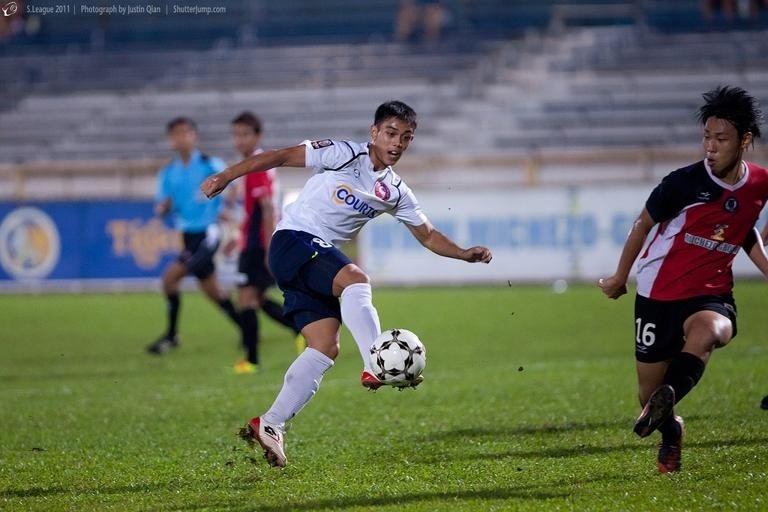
[657,415,684,473]
[360,370,424,394]
[633,384,675,438]
[244,415,293,468]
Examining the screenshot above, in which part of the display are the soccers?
[369,330,427,388]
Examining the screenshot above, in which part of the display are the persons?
[223,110,307,375]
[144,116,241,355]
[198,100,492,469]
[597,84,768,476]
[758,218,768,411]
